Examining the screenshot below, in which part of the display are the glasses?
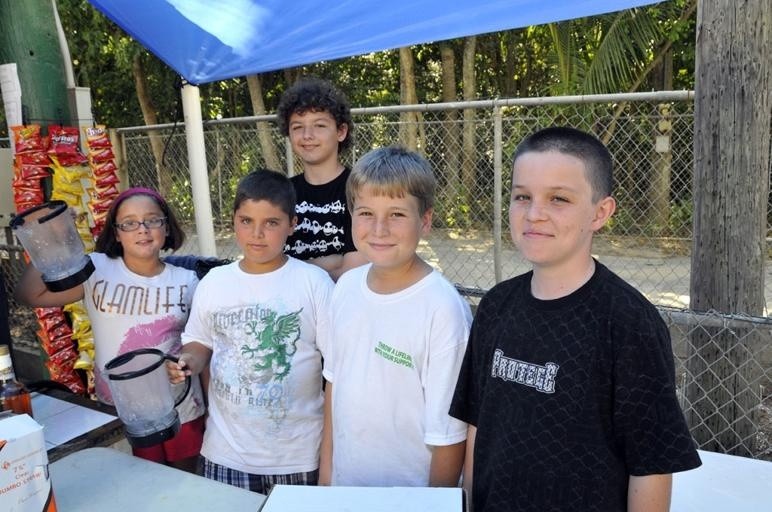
[113,216,168,232]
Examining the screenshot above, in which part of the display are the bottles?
[1,379,32,417]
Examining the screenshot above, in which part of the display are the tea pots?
[8,200,96,293]
[100,348,192,446]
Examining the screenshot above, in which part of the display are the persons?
[447,127,704,512]
[273,75,374,285]
[311,144,477,487]
[10,184,213,472]
[164,165,338,500]
